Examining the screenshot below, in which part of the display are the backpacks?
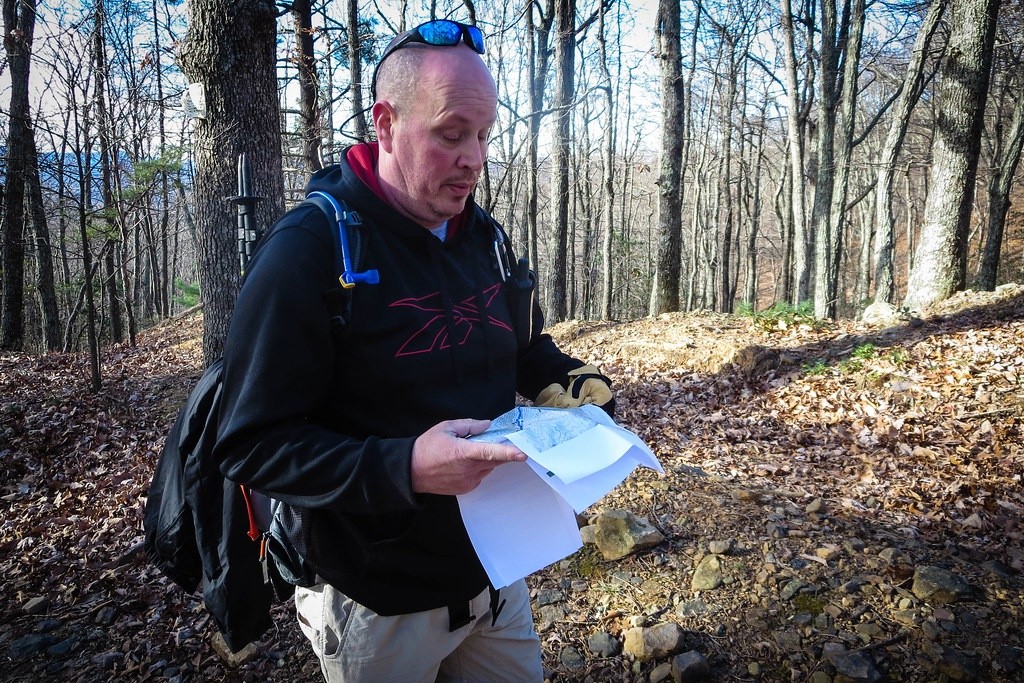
[241,189,512,602]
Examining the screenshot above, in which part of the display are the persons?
[215,18,619,683]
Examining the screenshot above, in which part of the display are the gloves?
[534,365,616,421]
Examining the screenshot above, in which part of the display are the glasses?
[371,19,485,93]
